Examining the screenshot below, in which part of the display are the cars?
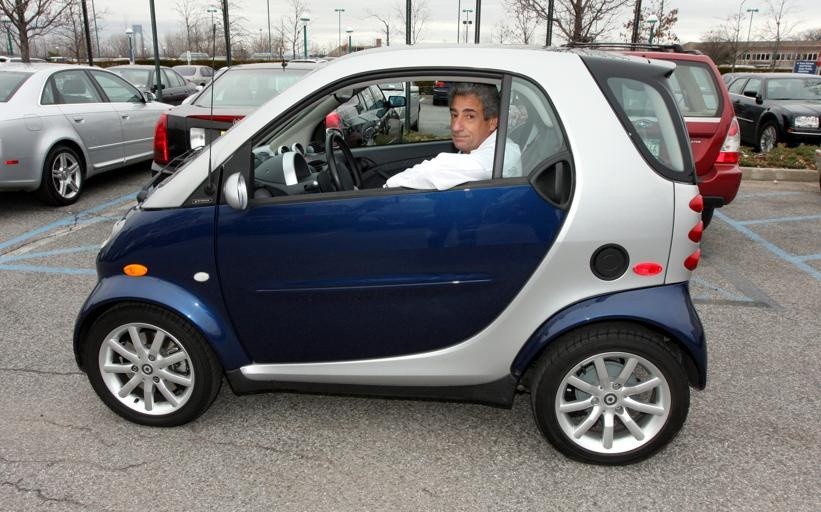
[73,43,706,465]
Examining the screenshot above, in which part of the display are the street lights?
[646,14,658,47]
[206,8,217,25]
[0,19,12,55]
[124,29,133,58]
[299,18,310,58]
[462,9,472,43]
[334,9,353,56]
[743,8,759,66]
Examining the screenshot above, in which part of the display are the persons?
[383,83,522,191]
